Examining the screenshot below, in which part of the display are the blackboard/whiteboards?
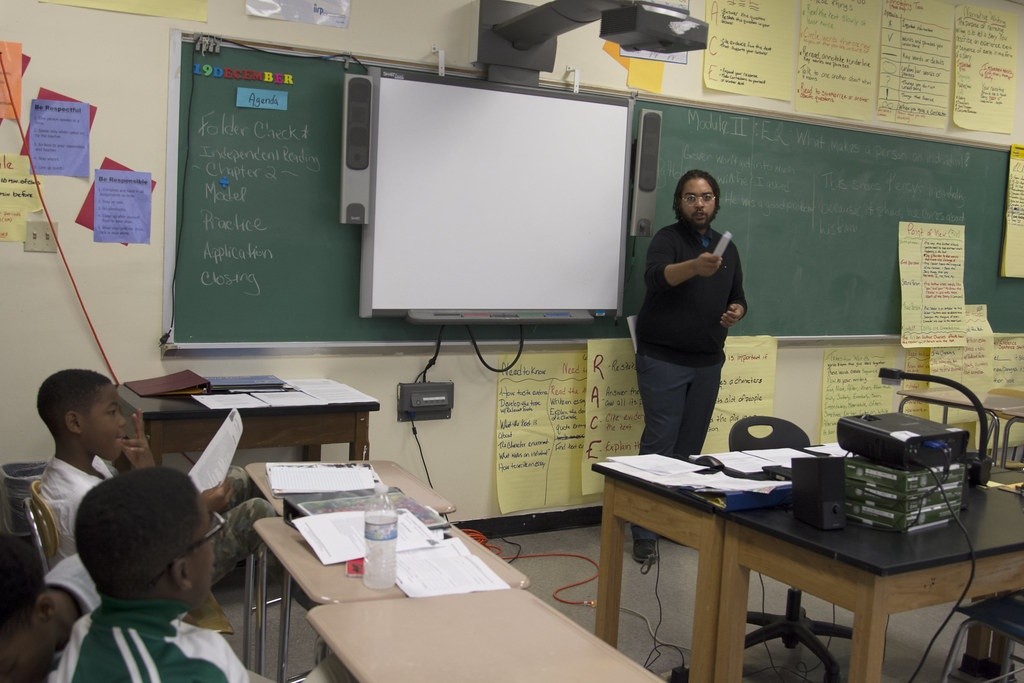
[161,28,1024,357]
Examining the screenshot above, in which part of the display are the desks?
[1000,406,1024,471]
[713,469,1024,683]
[303,585,668,683]
[252,511,533,678]
[239,458,454,676]
[110,374,380,466]
[896,385,1024,467]
[585,438,849,683]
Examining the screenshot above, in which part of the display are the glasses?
[153,510,227,584]
[681,194,716,206]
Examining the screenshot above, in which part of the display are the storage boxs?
[844,499,962,529]
[844,457,966,492]
[843,478,963,512]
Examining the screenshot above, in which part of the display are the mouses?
[695,455,725,468]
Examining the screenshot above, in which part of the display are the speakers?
[340,73,373,225]
[630,109,663,236]
[791,457,846,530]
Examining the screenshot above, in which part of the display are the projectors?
[837,411,969,472]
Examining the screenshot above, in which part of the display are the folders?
[283,486,452,530]
[679,485,792,512]
[123,369,211,397]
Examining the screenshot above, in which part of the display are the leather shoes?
[632,539,657,561]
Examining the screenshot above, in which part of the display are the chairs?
[19,477,58,574]
[939,587,1024,683]
[720,407,854,683]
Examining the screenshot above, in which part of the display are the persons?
[631,169,748,562]
[55,467,250,683]
[37,368,275,588]
[0,534,100,683]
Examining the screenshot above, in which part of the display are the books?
[282,487,451,529]
[205,375,285,390]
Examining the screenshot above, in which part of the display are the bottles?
[364,486,397,591]
